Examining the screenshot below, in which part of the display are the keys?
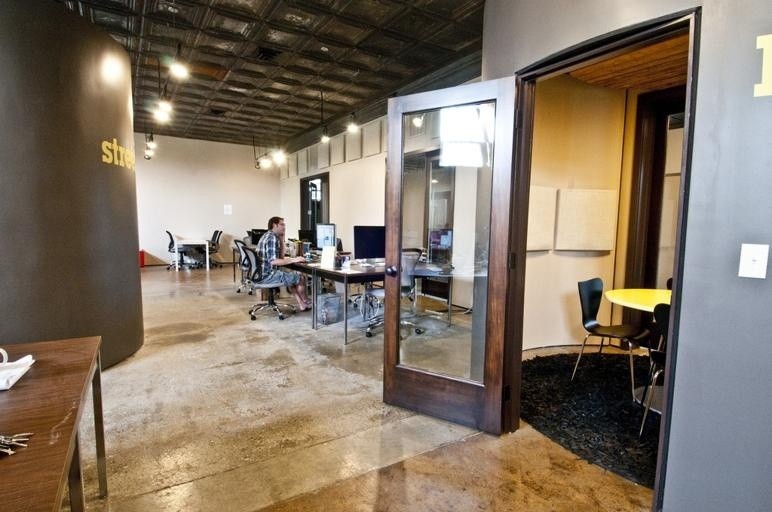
[0,432,36,455]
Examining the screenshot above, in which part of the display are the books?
[320,245,336,270]
[0,355,35,391]
[285,237,310,258]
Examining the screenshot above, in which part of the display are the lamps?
[253,60,360,169]
[143,0,190,160]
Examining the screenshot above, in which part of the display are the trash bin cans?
[316,293,341,325]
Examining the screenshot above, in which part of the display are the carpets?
[520,352,666,490]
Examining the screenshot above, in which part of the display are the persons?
[256,217,311,312]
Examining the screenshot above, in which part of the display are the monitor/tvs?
[251,229,268,245]
[298,229,322,251]
[316,223,336,249]
[353,226,385,260]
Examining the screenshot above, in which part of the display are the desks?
[0,336,107,512]
[232,246,453,345]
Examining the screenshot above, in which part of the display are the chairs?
[166,230,224,270]
[571,277,673,437]
[234,226,421,341]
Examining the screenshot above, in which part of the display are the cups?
[290,248,296,258]
[294,241,311,257]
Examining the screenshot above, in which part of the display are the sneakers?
[300,299,312,311]
